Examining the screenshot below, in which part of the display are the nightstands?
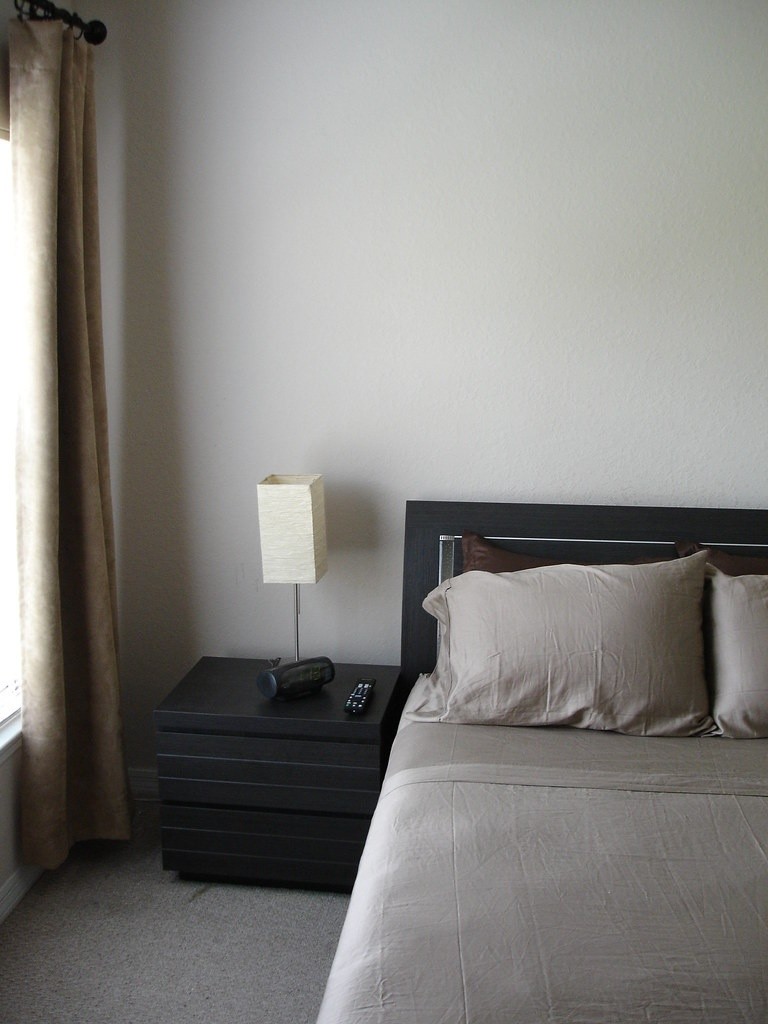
[152,654,404,892]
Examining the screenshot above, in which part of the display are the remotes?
[344,678,377,715]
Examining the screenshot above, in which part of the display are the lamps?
[259,469,330,705]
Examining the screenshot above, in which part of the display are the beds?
[313,498,768,1024]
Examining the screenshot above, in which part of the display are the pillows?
[703,562,768,742]
[406,548,722,739]
